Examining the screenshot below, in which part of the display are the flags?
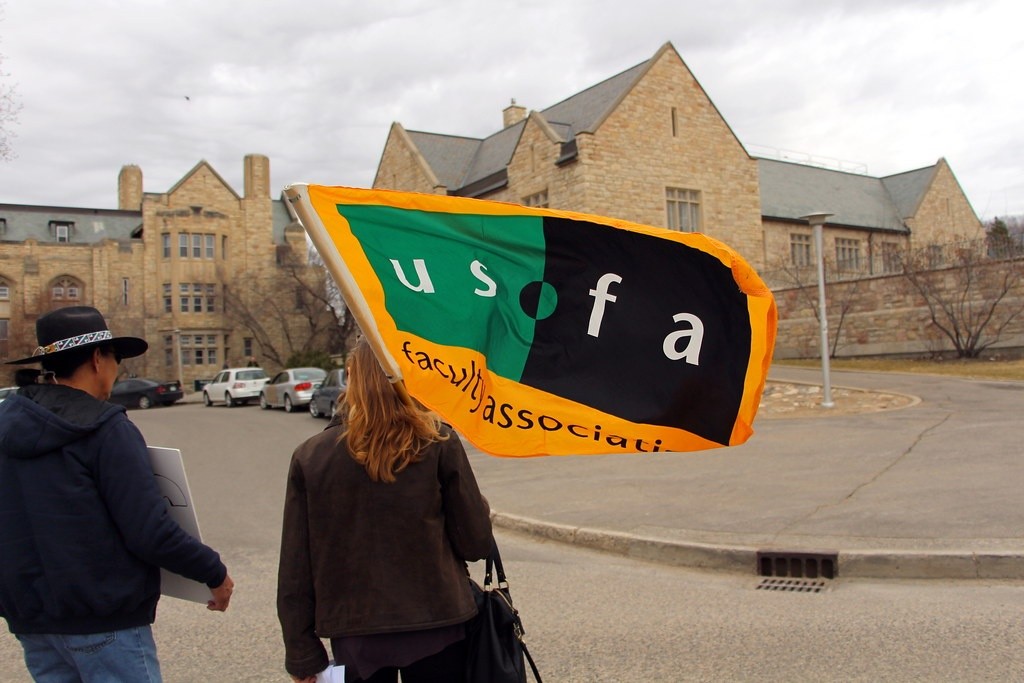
[292,184,777,459]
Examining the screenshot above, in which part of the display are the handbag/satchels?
[479,536,543,683]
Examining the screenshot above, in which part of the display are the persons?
[0,306,233,683]
[276,335,493,683]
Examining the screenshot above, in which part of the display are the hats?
[3,305,149,364]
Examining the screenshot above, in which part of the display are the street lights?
[798,211,836,408]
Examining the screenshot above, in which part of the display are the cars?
[258,366,327,413]
[0,387,20,404]
[308,369,348,419]
[108,378,185,410]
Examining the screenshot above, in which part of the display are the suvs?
[202,366,270,408]
